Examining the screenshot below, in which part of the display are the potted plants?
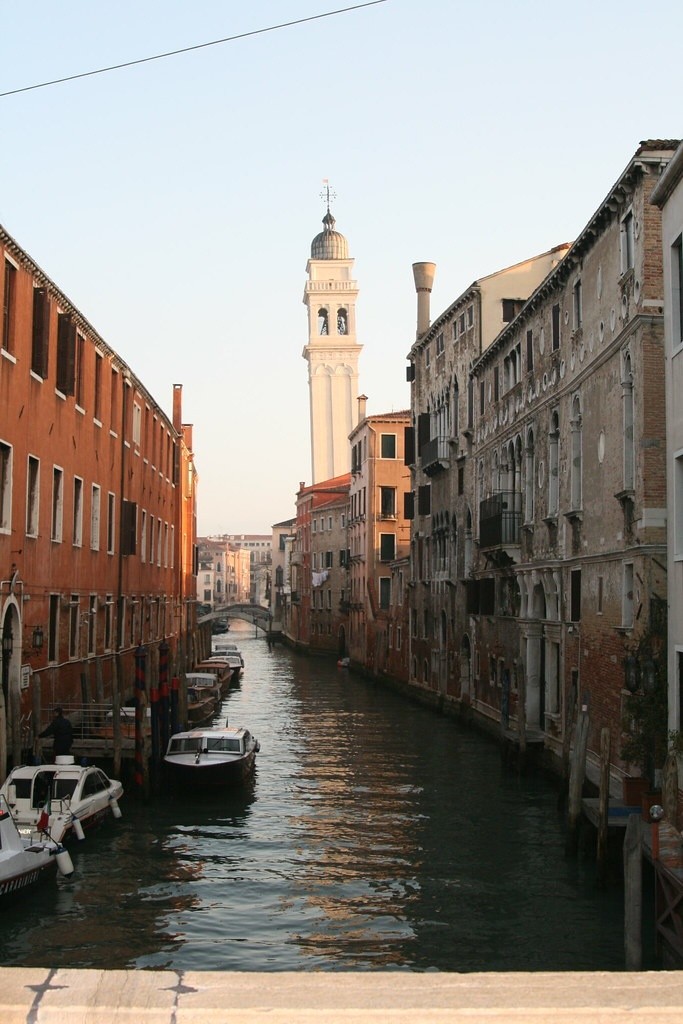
[621,592,668,824]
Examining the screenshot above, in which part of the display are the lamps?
[24,624,44,648]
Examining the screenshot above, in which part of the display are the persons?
[35,707,74,755]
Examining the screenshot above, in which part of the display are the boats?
[0,794,74,894]
[215,620,229,632]
[0,755,124,844]
[185,643,245,724]
[162,726,260,787]
